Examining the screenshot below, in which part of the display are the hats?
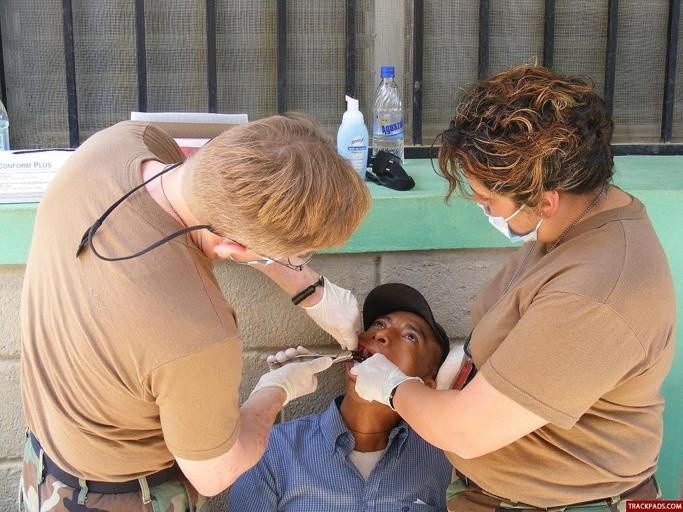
[362,283,449,359]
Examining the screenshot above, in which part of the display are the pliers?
[271,351,363,367]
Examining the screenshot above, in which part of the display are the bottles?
[0,100,10,151]
[372,66,403,167]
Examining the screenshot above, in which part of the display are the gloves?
[298,276,363,352]
[349,353,425,411]
[249,346,333,407]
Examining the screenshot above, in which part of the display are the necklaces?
[347,428,388,435]
[160,162,194,242]
[503,177,609,297]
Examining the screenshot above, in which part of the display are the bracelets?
[291,275,324,306]
[389,378,420,409]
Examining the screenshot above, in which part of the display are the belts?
[27,430,179,495]
[454,467,651,509]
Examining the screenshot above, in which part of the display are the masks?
[475,202,543,244]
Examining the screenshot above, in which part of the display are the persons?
[348,54,678,512]
[226,282,452,512]
[13,107,362,511]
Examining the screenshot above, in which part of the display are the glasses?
[217,233,315,272]
